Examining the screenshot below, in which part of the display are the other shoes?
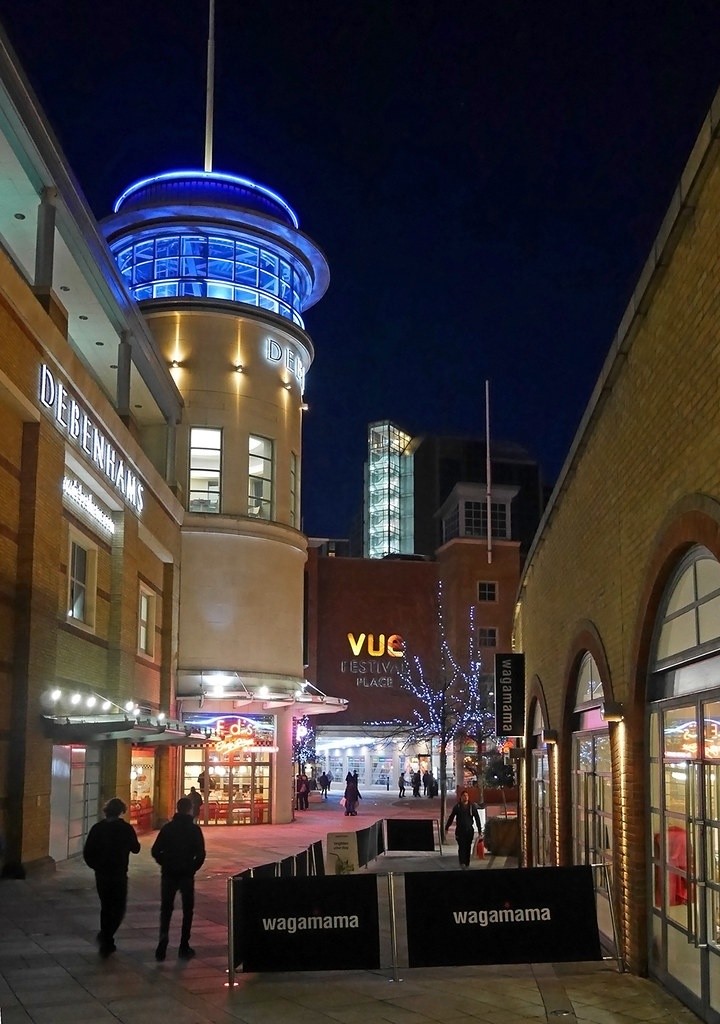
[466,866,470,869]
[402,796,406,797]
[462,864,466,869]
[156,938,169,961]
[344,811,357,816]
[179,943,194,956]
[399,796,403,798]
[300,784,306,792]
[98,929,116,953]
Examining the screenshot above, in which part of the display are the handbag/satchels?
[472,835,486,860]
[340,797,346,806]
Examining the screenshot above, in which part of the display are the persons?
[411,770,422,798]
[320,771,334,799]
[186,787,203,824]
[297,775,311,811]
[398,773,409,798]
[151,797,206,962]
[387,777,389,790]
[445,791,482,869]
[82,797,141,959]
[198,770,211,796]
[423,770,438,799]
[344,770,362,816]
